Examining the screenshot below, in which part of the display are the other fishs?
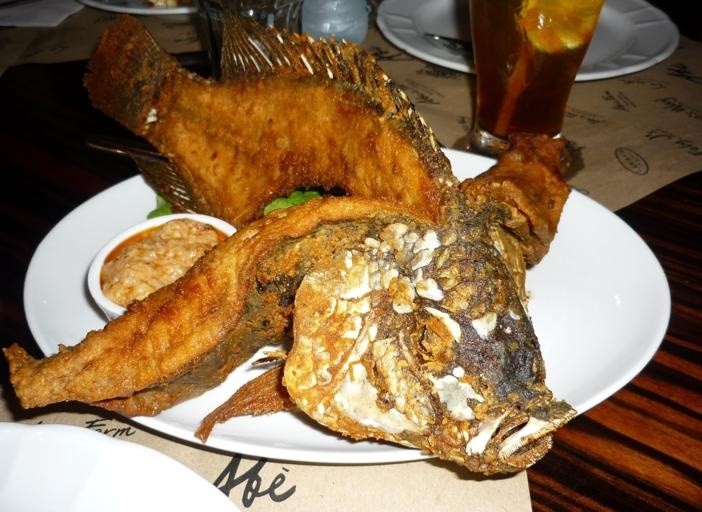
[2,0,585,477]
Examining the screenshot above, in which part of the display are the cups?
[457,0,606,160]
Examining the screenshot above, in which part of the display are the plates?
[21,144,671,468]
[84,1,196,17]
[1,420,240,512]
[372,0,681,84]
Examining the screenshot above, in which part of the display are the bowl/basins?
[85,213,240,325]
